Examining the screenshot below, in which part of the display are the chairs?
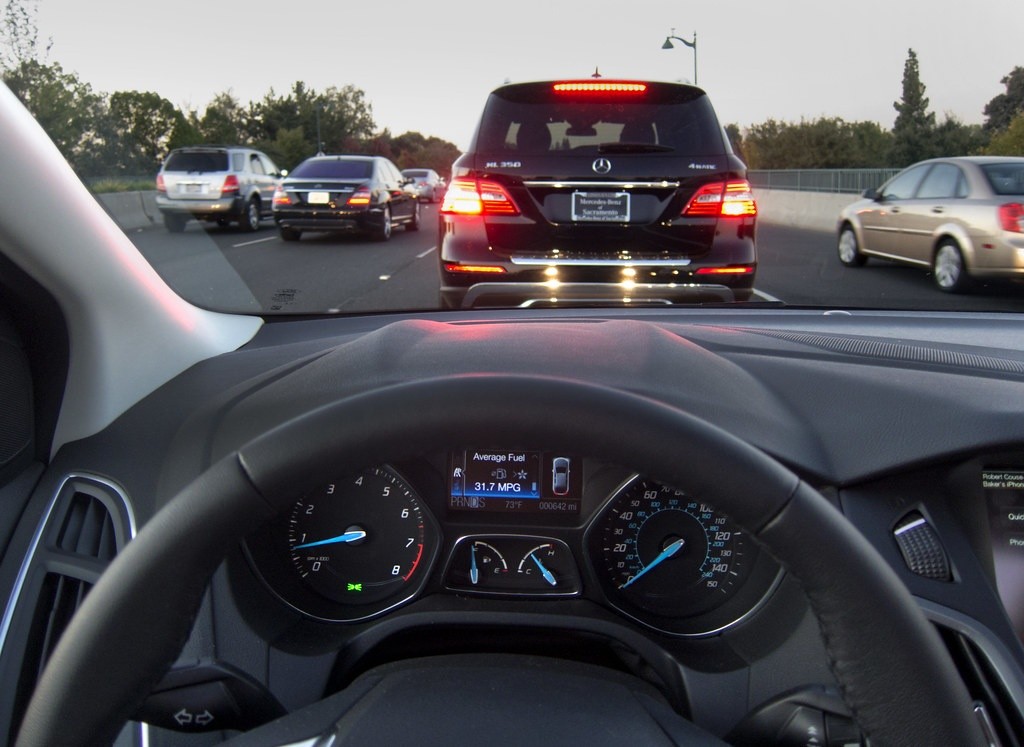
[619,121,657,145]
[515,121,551,152]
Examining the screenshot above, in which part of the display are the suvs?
[432,77,760,317]
[154,147,285,234]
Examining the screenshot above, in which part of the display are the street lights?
[663,26,699,86]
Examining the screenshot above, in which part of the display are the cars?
[270,153,421,240]
[400,167,446,204]
[835,154,1024,292]
